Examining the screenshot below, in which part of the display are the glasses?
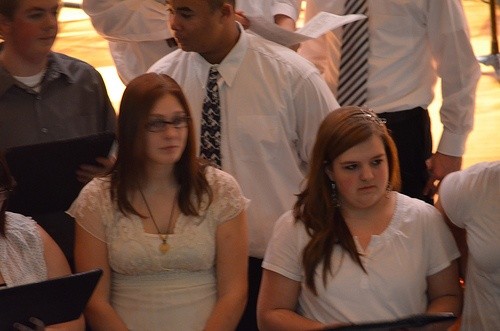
[146,116,192,132]
[0,190,12,201]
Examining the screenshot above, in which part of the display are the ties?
[336,0,369,108]
[200,67,222,171]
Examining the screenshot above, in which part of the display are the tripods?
[475,0,500,84]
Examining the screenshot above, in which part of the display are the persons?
[0,149,86,331]
[0,0,118,273]
[65,73,252,331]
[148,0,340,330]
[300,0,480,206]
[82,0,303,88]
[436,161,500,331]
[257,107,460,331]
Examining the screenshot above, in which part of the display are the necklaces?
[139,186,176,253]
